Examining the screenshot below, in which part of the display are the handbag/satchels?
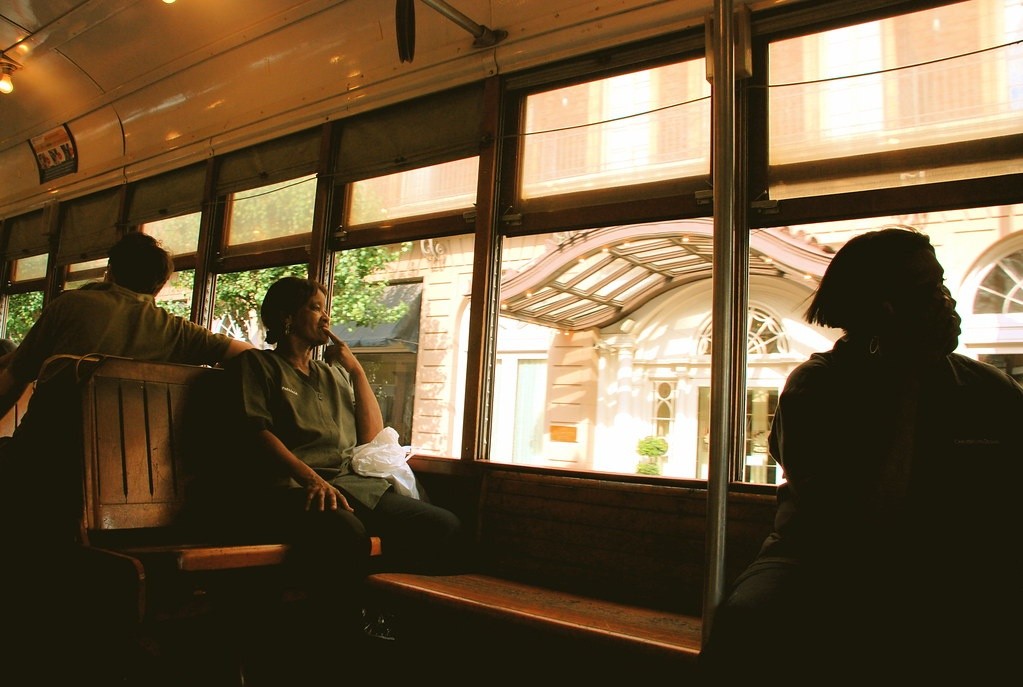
[351,426,419,501]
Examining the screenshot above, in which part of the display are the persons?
[0,233,253,458]
[0,338,15,356]
[696,229,1022,687]
[231,276,463,581]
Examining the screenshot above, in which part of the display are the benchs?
[15,352,383,634]
[861,355,1023,687]
[371,467,777,660]
